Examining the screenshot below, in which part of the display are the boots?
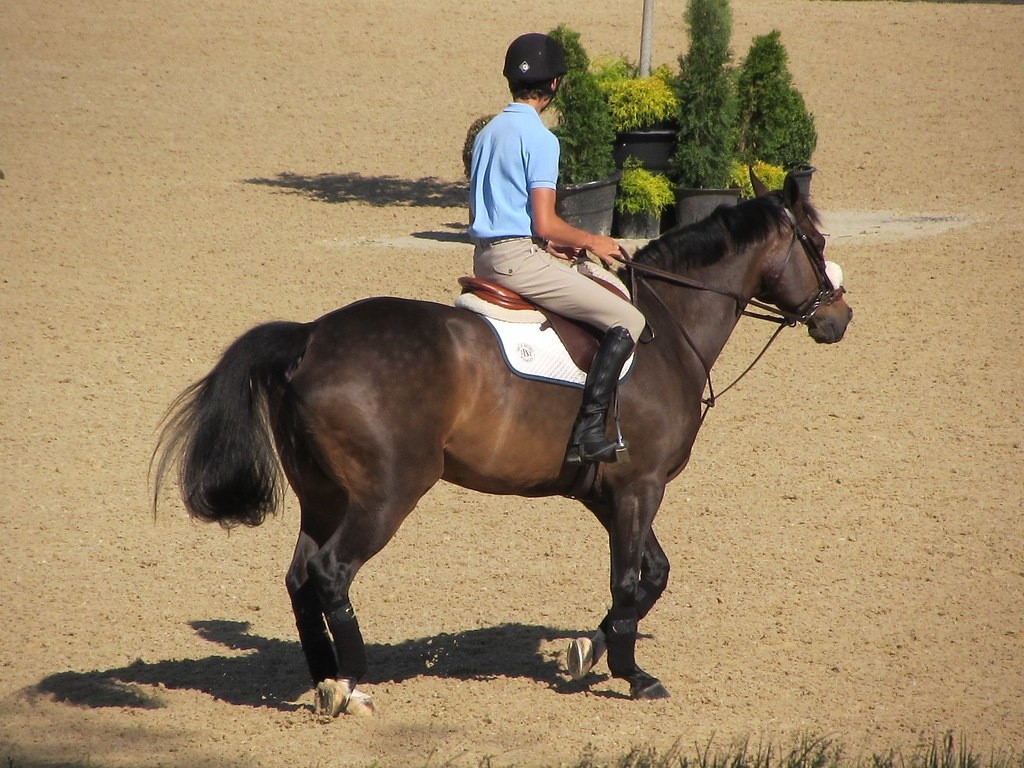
[565,326,630,468]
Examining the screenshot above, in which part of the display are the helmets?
[503,33,565,82]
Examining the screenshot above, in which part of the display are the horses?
[147,165,853,721]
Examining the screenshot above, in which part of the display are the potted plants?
[615,156,671,239]
[463,113,496,226]
[674,72,746,229]
[606,75,675,177]
[745,28,816,206]
[547,24,617,238]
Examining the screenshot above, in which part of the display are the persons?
[466,33,645,468]
